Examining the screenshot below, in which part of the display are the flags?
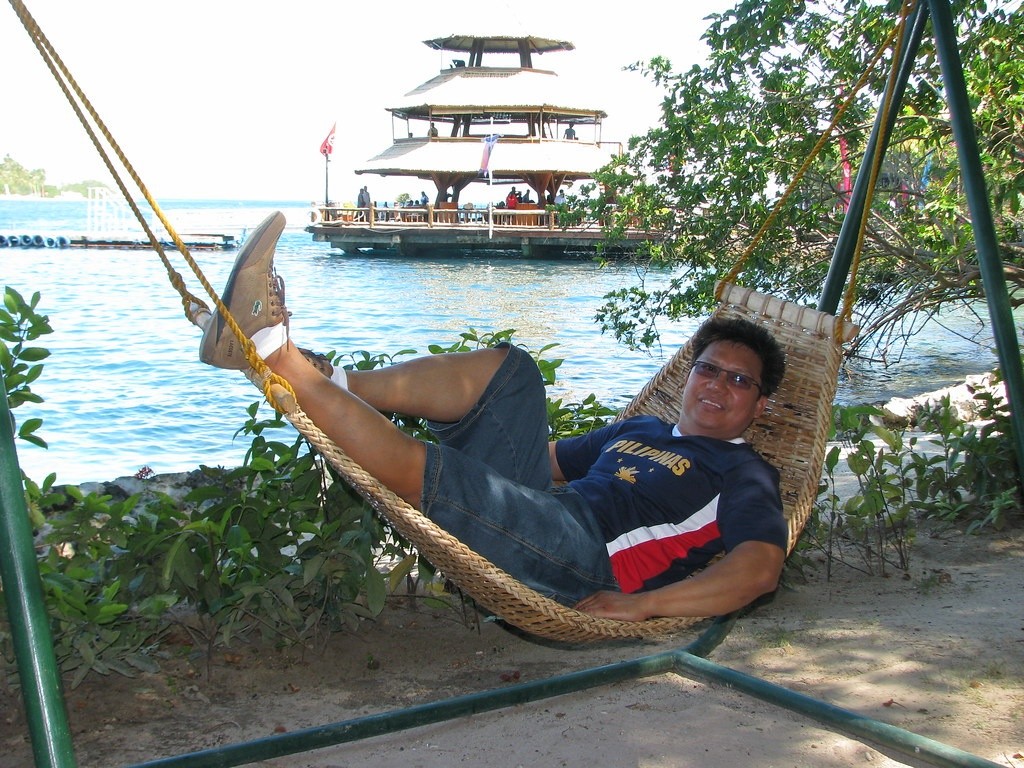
[319,121,337,155]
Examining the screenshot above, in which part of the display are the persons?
[201,212,789,621]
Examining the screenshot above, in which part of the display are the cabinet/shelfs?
[438,202,458,223]
[512,203,539,226]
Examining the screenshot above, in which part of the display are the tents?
[355,18,625,227]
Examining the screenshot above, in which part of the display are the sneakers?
[297,347,334,379]
[199,212,287,370]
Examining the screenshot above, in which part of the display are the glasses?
[690,361,762,391]
